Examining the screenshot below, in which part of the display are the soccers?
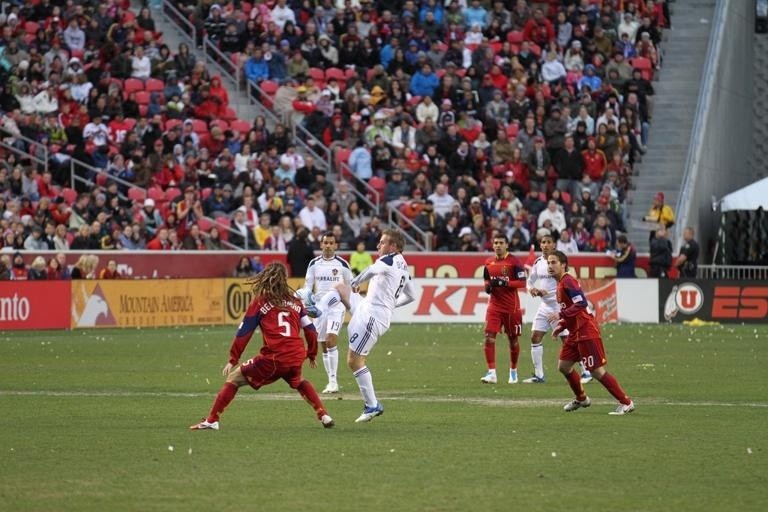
[293,288,317,307]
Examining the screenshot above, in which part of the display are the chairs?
[632,57,653,83]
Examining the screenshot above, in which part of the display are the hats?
[458,227,472,237]
[318,34,331,43]
[143,199,155,209]
[654,192,664,202]
[69,57,80,66]
[279,39,289,47]
[571,41,582,49]
[7,13,18,25]
[211,4,221,11]
[502,171,515,179]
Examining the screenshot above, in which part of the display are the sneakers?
[190,419,220,431]
[321,414,334,428]
[480,375,498,384]
[563,395,591,411]
[305,306,322,318]
[509,370,518,384]
[322,383,339,394]
[608,399,634,416]
[354,401,384,423]
[580,374,593,384]
[522,375,545,384]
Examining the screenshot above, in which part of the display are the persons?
[1,1,406,281]
[546,250,635,415]
[301,232,356,397]
[522,233,593,384]
[286,1,676,281]
[188,262,336,430]
[317,229,417,424]
[674,226,701,278]
[479,234,527,385]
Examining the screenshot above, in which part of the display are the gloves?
[492,278,505,287]
